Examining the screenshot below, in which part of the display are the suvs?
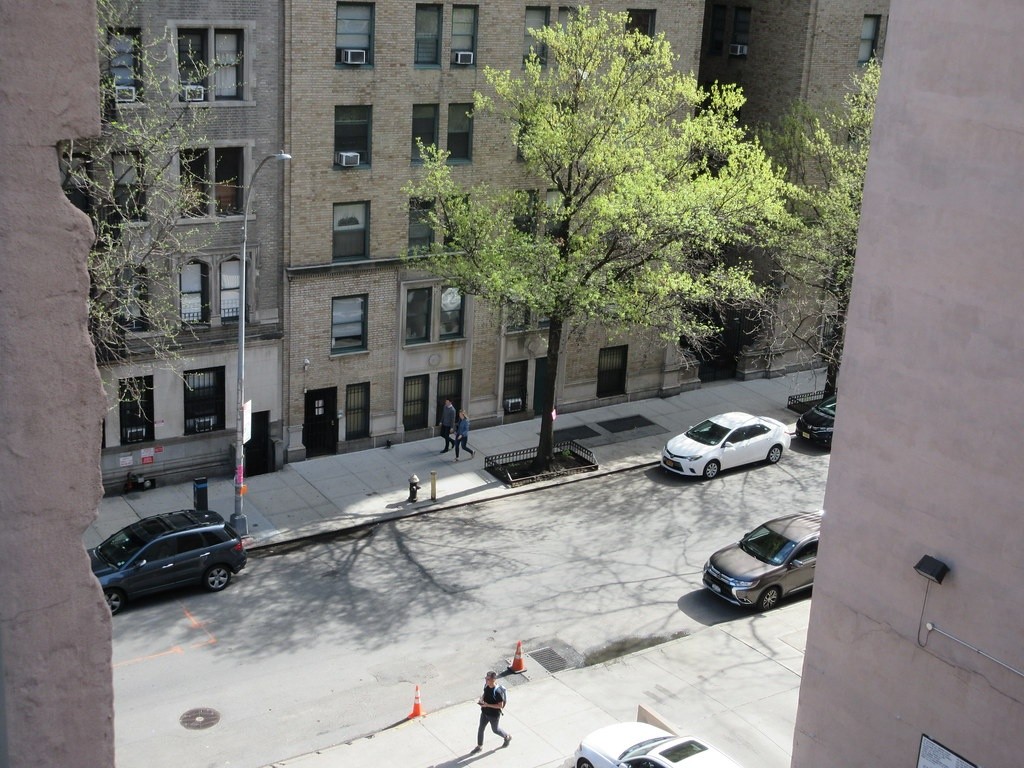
[86,509,247,615]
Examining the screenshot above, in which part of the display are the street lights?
[229,150,292,536]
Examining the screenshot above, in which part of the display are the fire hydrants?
[408,473,421,502]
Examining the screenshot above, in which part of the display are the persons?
[454,408,475,461]
[470,671,512,753]
[438,398,455,453]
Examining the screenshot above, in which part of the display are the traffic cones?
[409,685,427,719]
[508,640,527,674]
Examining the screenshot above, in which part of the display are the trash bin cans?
[193,477,208,510]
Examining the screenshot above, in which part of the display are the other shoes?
[471,746,482,754]
[472,450,475,457]
[440,450,448,453]
[502,736,512,748]
[452,441,455,448]
[453,458,459,461]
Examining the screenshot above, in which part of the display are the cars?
[703,510,822,611]
[575,722,743,768]
[661,412,791,479]
[796,395,837,450]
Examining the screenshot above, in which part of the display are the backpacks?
[485,683,507,708]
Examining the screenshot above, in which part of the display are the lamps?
[914,554,947,585]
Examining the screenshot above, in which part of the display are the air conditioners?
[342,49,366,65]
[194,419,212,432]
[181,85,204,101]
[115,86,136,104]
[454,51,473,64]
[506,397,522,412]
[337,152,359,166]
[126,426,145,442]
[729,44,748,55]
